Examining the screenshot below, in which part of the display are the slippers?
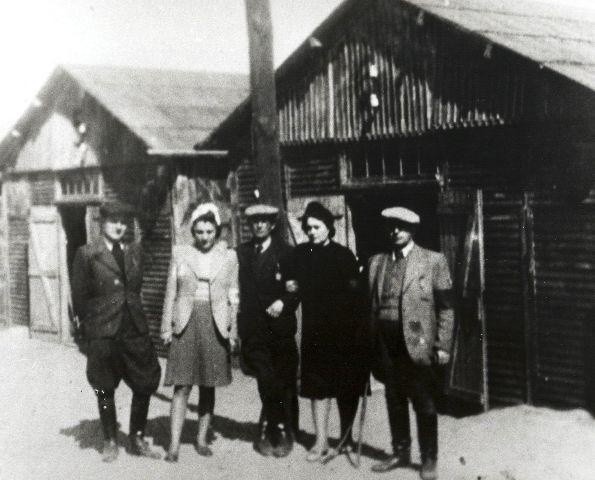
[307,439,329,461]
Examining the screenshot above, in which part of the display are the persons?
[281,200,372,463]
[234,204,302,459]
[72,202,164,465]
[157,201,241,461]
[363,207,456,480]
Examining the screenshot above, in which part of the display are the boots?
[372,401,412,473]
[98,396,118,462]
[256,412,276,456]
[126,395,160,458]
[274,413,293,457]
[415,406,438,479]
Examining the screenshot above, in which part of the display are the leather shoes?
[194,437,214,456]
[164,453,179,463]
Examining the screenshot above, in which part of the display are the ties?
[112,243,126,274]
[257,242,263,253]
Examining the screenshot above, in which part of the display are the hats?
[382,205,420,226]
[244,204,279,216]
[297,201,342,224]
[101,201,136,217]
